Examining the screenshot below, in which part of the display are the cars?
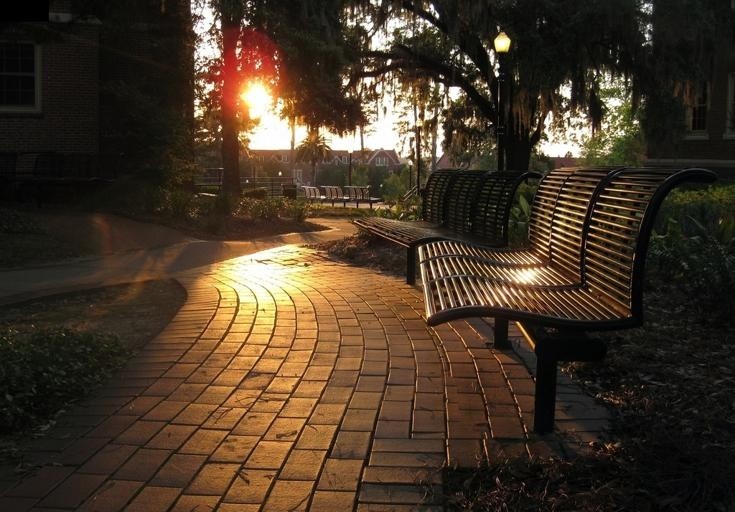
[199,166,224,182]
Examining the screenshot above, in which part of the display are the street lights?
[492,28,515,171]
[347,145,354,185]
[245,179,248,189]
[278,170,283,188]
[380,183,383,198]
[408,159,414,189]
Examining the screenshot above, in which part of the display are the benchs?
[299,184,382,210]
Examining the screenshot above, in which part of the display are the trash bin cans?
[281,184,297,200]
